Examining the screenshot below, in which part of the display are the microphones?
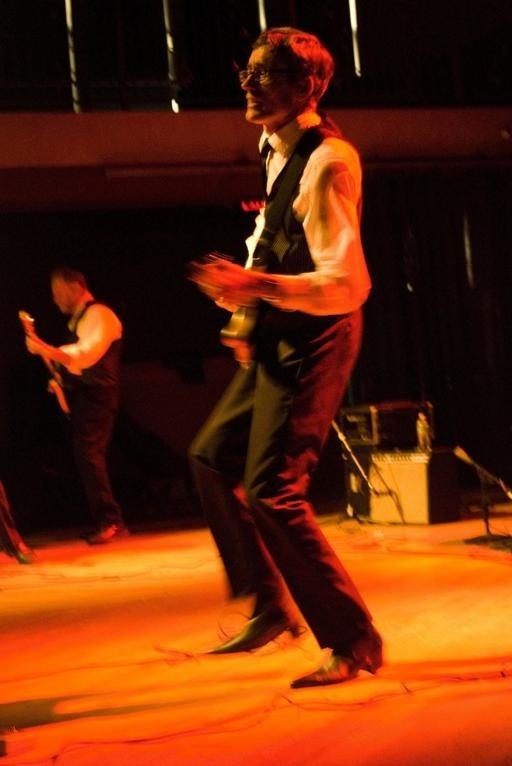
[452,445,499,484]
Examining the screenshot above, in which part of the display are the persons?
[189,27,384,688]
[25,267,130,545]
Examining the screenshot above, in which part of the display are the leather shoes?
[207,601,306,655]
[85,522,130,540]
[290,632,388,687]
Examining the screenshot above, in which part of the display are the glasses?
[238,67,269,84]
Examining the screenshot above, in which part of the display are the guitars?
[193,264,267,369]
[18,311,70,414]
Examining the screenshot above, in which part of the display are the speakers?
[343,452,430,524]
